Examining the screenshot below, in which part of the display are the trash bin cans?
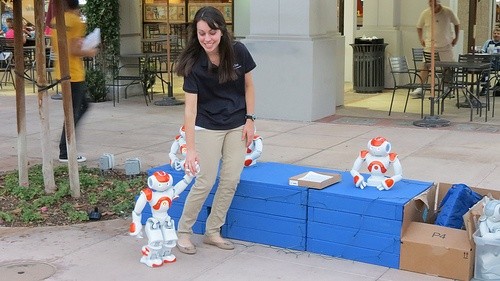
[350,37,388,93]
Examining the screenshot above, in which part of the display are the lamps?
[123,157,142,177]
[98,153,115,171]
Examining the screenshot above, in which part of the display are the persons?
[350,136,403,191]
[49,0,99,162]
[410,0,460,98]
[0,17,27,46]
[477,200,500,281]
[177,6,256,254]
[244,125,263,168]
[479,28,500,97]
[130,160,201,269]
[170,121,188,171]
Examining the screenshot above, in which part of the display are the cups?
[487,43,494,55]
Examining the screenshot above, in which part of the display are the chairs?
[144,24,183,73]
[459,54,500,121]
[423,51,456,96]
[114,53,151,106]
[388,56,440,118]
[0,36,57,93]
[410,48,440,99]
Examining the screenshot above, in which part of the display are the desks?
[119,52,178,99]
[429,62,491,120]
[142,39,166,50]
[154,34,176,47]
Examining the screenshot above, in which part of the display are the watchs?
[244,114,256,121]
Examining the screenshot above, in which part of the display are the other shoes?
[203,236,235,250]
[409,88,425,97]
[480,87,488,96]
[443,92,454,97]
[176,242,196,254]
[59,156,86,162]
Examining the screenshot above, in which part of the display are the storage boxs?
[289,170,341,189]
[471,229,500,281]
[400,182,499,281]
